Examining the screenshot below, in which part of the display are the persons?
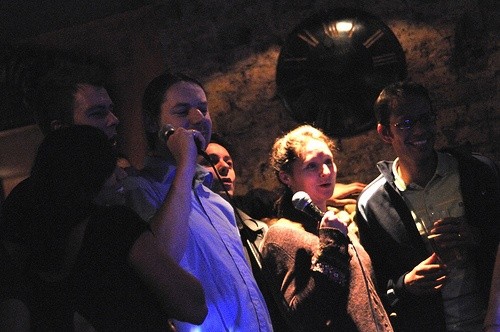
[0,78,207,332]
[356,82,500,332]
[123,71,273,332]
[260,122,395,332]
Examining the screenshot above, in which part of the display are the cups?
[420,207,462,268]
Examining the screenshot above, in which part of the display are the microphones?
[159,124,204,155]
[292,191,352,244]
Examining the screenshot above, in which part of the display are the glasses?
[381,111,441,130]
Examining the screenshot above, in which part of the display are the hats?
[6,124,118,226]
